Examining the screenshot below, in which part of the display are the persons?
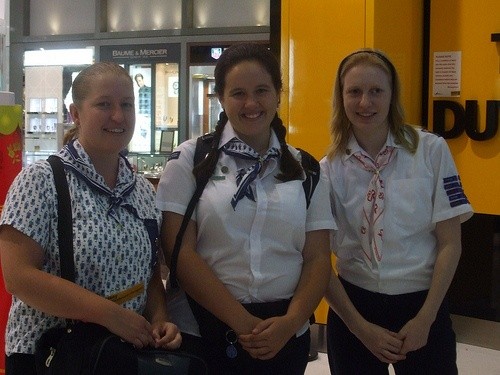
[135,73,152,114]
[3,62,183,374]
[315,49,474,375]
[156,42,339,375]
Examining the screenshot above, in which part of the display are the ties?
[225,138,279,212]
[352,146,397,265]
[51,142,136,221]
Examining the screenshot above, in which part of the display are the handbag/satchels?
[138,346,209,375]
[36,323,138,375]
[166,272,202,338]
